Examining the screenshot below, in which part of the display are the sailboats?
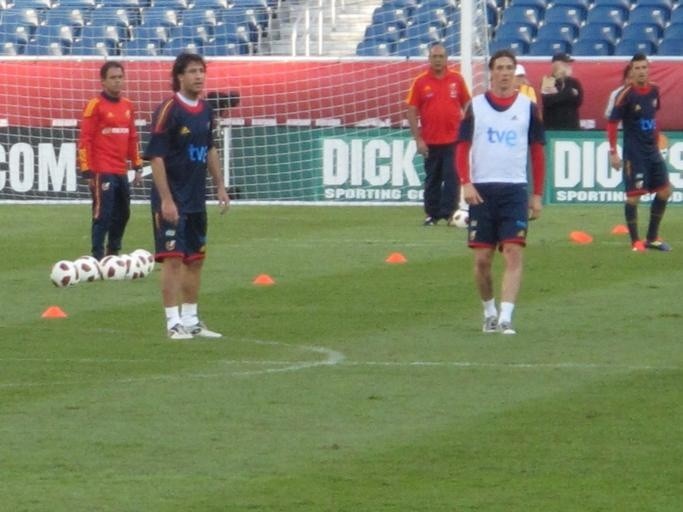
[50,249,155,287]
[452,209,469,228]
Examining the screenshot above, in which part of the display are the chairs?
[482,316,499,333]
[422,216,437,226]
[497,321,516,334]
[167,322,222,339]
[642,237,671,251]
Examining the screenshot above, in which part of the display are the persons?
[606,52,673,254]
[604,61,633,130]
[77,61,142,260]
[455,49,546,334]
[514,63,538,123]
[406,45,471,226]
[540,51,583,132]
[142,53,230,339]
[205,90,240,119]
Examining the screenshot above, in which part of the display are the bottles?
[514,63,525,76]
[551,51,574,63]
